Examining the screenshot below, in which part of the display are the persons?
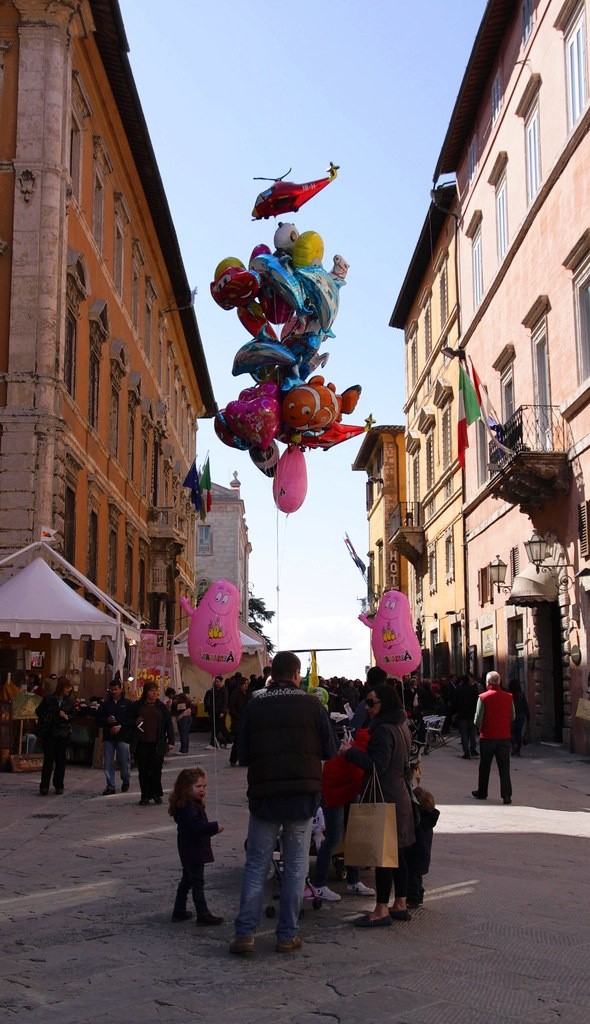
[229,652,338,954]
[202,667,530,908]
[168,767,226,925]
[33,678,76,797]
[338,679,418,928]
[94,680,135,795]
[132,681,175,806]
[164,686,192,756]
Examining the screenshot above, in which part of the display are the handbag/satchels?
[343,760,399,868]
[32,720,47,737]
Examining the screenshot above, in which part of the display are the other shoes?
[175,751,188,756]
[206,745,216,750]
[354,913,392,926]
[226,743,234,748]
[472,791,487,800]
[171,911,192,923]
[504,798,511,804]
[197,914,224,924]
[103,788,115,795]
[139,796,150,806]
[152,796,163,804]
[389,908,411,921]
[121,783,129,792]
[39,789,48,794]
[55,789,63,795]
[406,901,424,908]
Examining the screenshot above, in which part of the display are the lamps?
[446,607,465,617]
[368,476,383,484]
[522,529,573,573]
[20,167,35,201]
[487,554,511,594]
[441,348,463,361]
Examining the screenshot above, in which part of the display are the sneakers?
[345,881,375,896]
[276,939,302,952]
[313,886,341,901]
[230,936,255,952]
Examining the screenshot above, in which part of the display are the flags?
[471,362,493,425]
[201,457,214,513]
[184,460,202,512]
[457,362,482,471]
[344,538,368,584]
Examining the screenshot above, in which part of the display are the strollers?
[265,835,322,920]
[407,712,431,755]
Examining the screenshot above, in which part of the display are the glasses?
[365,699,384,708]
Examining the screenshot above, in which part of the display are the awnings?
[503,554,559,610]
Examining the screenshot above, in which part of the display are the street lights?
[442,346,469,672]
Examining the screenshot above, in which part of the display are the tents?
[0,556,141,684]
[172,629,263,717]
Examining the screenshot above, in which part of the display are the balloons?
[358,590,422,680]
[180,579,243,675]
[209,162,377,515]
